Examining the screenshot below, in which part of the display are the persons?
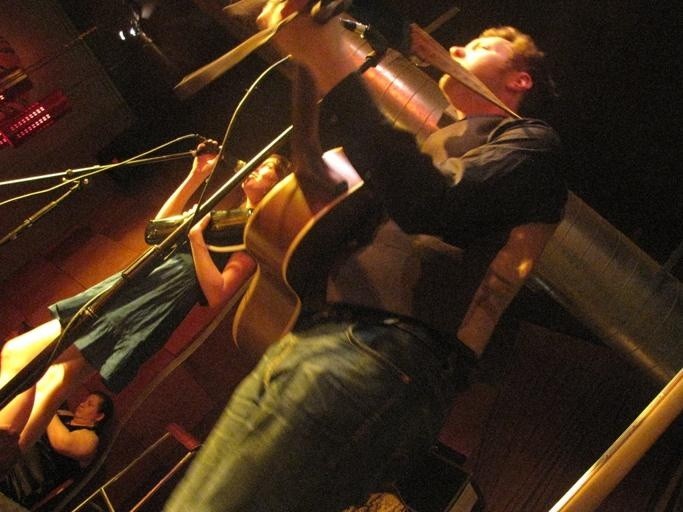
[1,391,113,508]
[1,139,295,454]
[160,0,567,512]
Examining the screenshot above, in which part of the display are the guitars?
[231,1,391,359]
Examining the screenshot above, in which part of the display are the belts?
[308,301,451,370]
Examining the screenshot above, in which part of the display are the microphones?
[195,133,248,173]
[342,16,431,69]
